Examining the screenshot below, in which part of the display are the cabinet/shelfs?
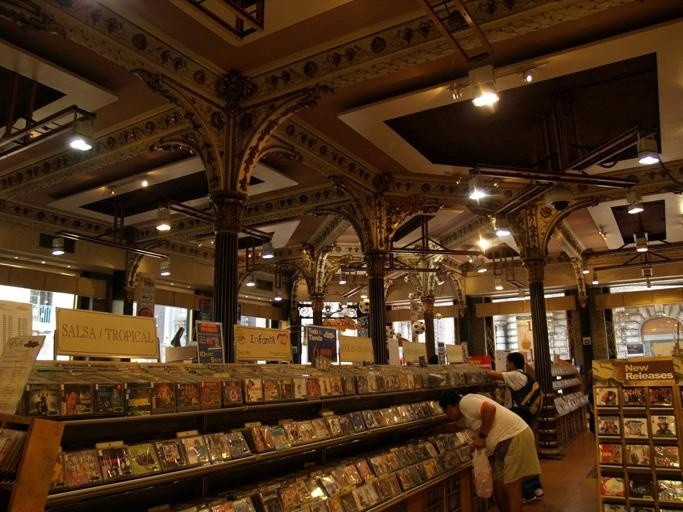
[46,382,514,512]
[592,386,683,512]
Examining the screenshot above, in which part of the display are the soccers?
[436,313,442,319]
[408,293,415,299]
[359,299,370,313]
[386,325,394,338]
[413,322,426,334]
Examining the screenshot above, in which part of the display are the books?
[0,363,489,512]
[594,387,683,512]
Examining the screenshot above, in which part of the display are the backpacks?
[506,370,544,418]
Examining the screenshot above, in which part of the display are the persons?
[584,367,596,439]
[426,391,543,512]
[490,352,544,504]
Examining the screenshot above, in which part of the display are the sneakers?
[522,487,545,504]
